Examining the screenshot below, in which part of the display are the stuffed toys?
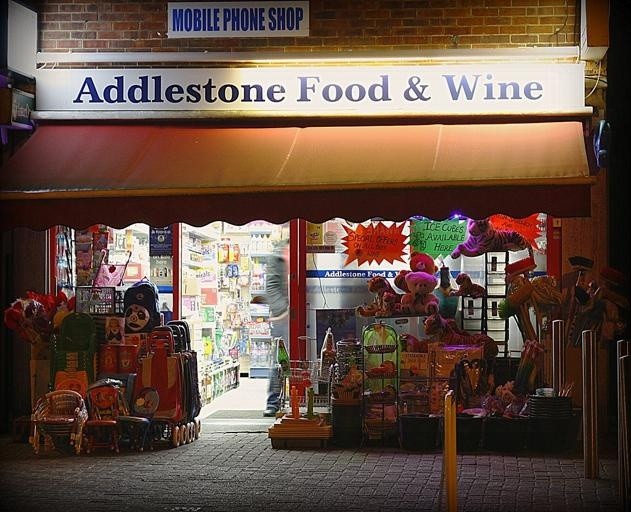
[394,252,439,292]
[401,271,440,315]
[451,218,524,259]
[451,270,484,298]
[356,276,402,317]
[375,292,401,317]
[420,312,499,361]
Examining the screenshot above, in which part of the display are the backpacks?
[123,277,161,334]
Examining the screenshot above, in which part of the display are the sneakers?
[261,406,280,416]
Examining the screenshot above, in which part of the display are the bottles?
[263,234,268,253]
[257,234,263,254]
[277,339,291,375]
[251,234,257,254]
[307,387,315,420]
[321,334,336,379]
[232,243,240,261]
[290,385,300,419]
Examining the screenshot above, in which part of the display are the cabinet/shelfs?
[242,228,285,378]
[361,319,400,452]
[398,353,429,417]
[181,222,241,406]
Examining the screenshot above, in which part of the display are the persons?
[265,238,289,418]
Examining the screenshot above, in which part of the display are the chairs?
[30,390,85,457]
[48,310,97,389]
[86,386,121,453]
[116,383,153,452]
